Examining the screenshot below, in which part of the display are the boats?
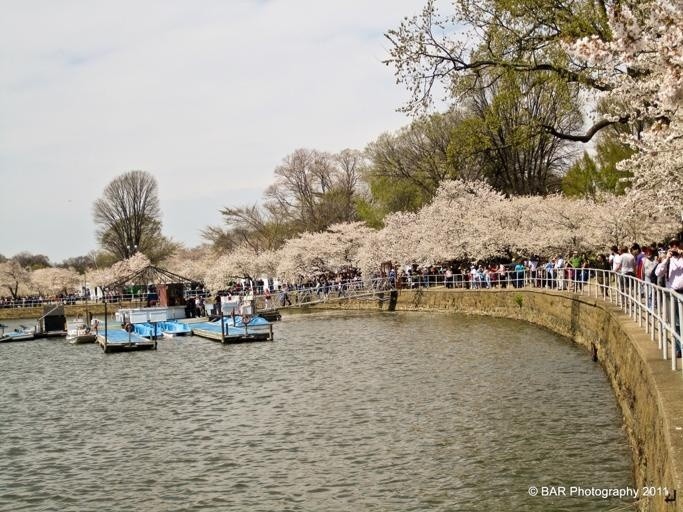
[214,315,273,330]
[130,320,193,338]
[64,330,97,345]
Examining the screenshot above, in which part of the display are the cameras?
[671,249,679,256]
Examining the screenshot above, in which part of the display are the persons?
[403,237,682,360]
[0,264,403,316]
